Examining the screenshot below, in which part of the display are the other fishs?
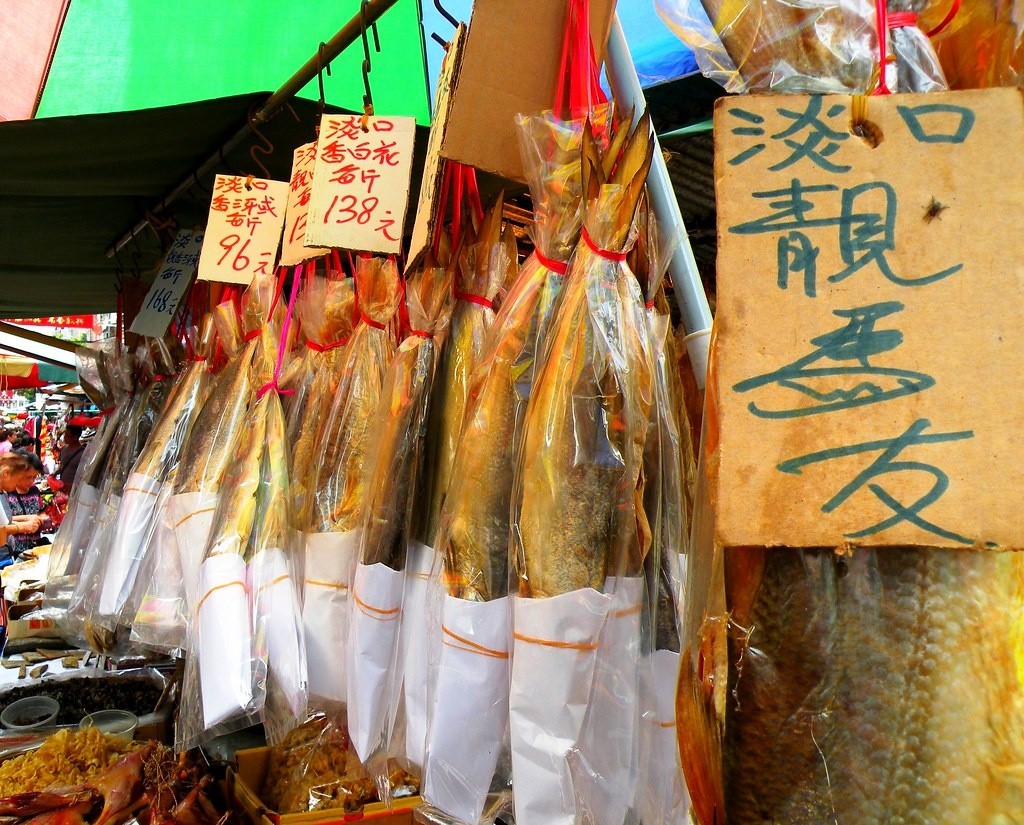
[46,99,693,652]
[695,0,1024,825]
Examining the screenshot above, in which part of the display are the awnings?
[0,352,82,391]
[1,0,768,134]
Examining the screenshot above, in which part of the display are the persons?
[0,452,41,572]
[1,416,47,476]
[5,450,56,557]
[46,425,88,495]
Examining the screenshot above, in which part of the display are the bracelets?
[14,523,20,535]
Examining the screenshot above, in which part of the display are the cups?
[79,710,139,742]
[1,696,60,732]
